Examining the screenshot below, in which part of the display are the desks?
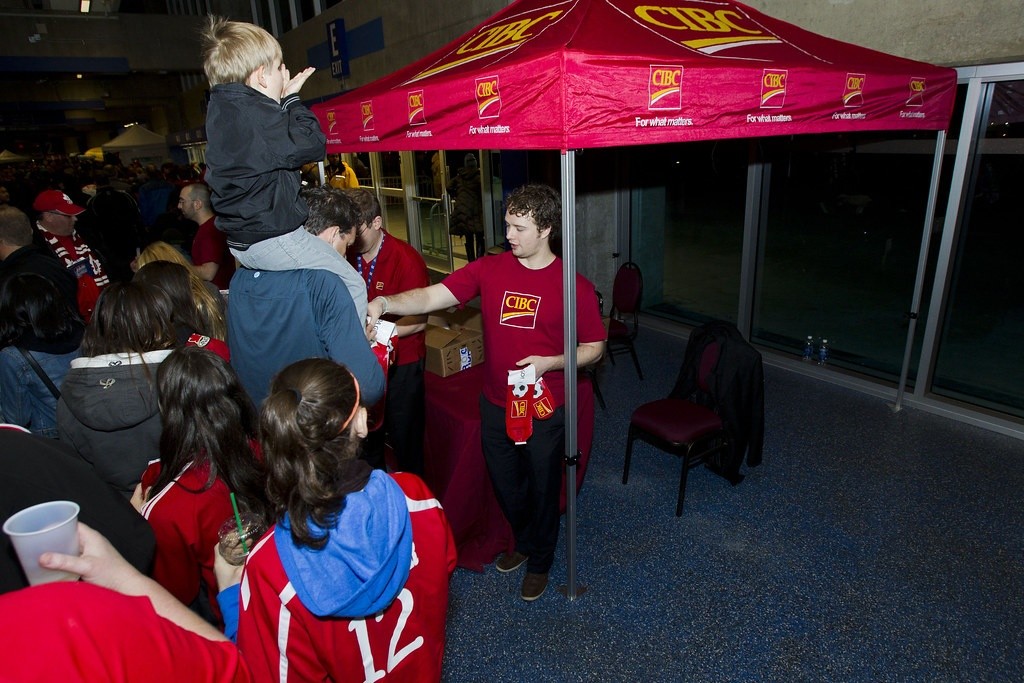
[422,369,595,572]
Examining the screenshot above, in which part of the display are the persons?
[0,15,608,682]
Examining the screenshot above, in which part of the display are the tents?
[308,1,958,601]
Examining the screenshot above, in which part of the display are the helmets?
[352,152,357,158]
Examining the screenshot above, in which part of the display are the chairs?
[603,262,643,380]
[623,320,764,518]
[582,290,607,411]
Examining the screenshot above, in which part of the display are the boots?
[465,242,475,263]
[476,238,485,258]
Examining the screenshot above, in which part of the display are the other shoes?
[520,571,549,601]
[496,548,529,573]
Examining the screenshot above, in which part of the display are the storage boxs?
[421,305,484,377]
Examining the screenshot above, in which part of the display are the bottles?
[802,336,815,362]
[817,338,832,366]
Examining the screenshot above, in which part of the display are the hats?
[464,153,478,170]
[34,190,85,217]
[167,159,174,163]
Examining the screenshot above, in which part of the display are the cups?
[218,512,269,565]
[3,500,81,587]
